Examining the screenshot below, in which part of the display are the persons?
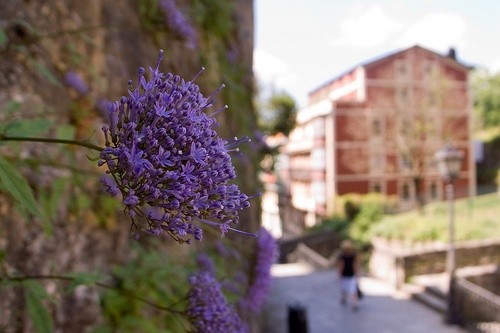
[334,240,362,312]
